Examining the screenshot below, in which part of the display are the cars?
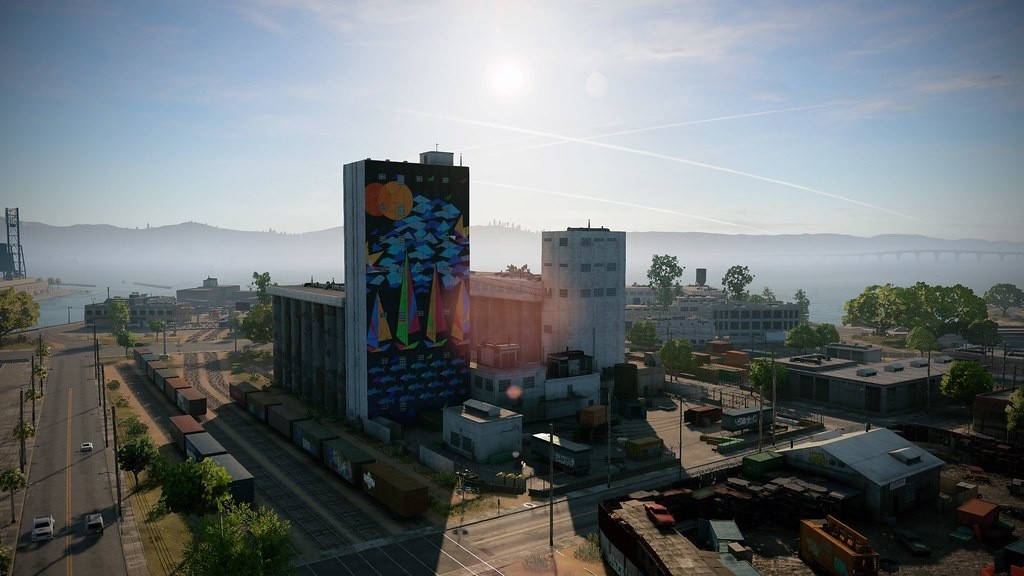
[80,441,93,452]
[84,513,104,536]
[30,514,56,543]
[644,502,676,528]
